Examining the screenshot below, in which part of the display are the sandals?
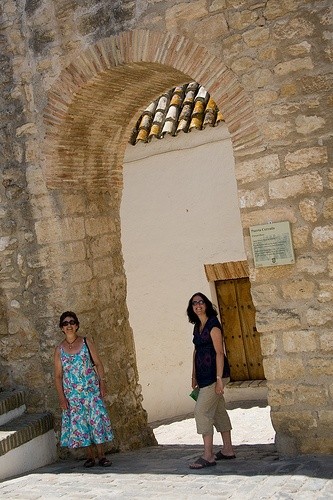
[83,458,95,468]
[98,458,112,467]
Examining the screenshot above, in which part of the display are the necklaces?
[65,336,78,349]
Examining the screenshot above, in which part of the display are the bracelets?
[216,376,222,379]
[192,377,196,379]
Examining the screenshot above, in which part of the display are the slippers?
[189,457,217,469]
[213,449,236,461]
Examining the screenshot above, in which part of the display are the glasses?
[59,320,77,326]
[192,300,205,306]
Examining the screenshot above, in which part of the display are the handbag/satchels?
[83,337,101,381]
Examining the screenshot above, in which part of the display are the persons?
[54,311,114,466]
[186,292,236,469]
